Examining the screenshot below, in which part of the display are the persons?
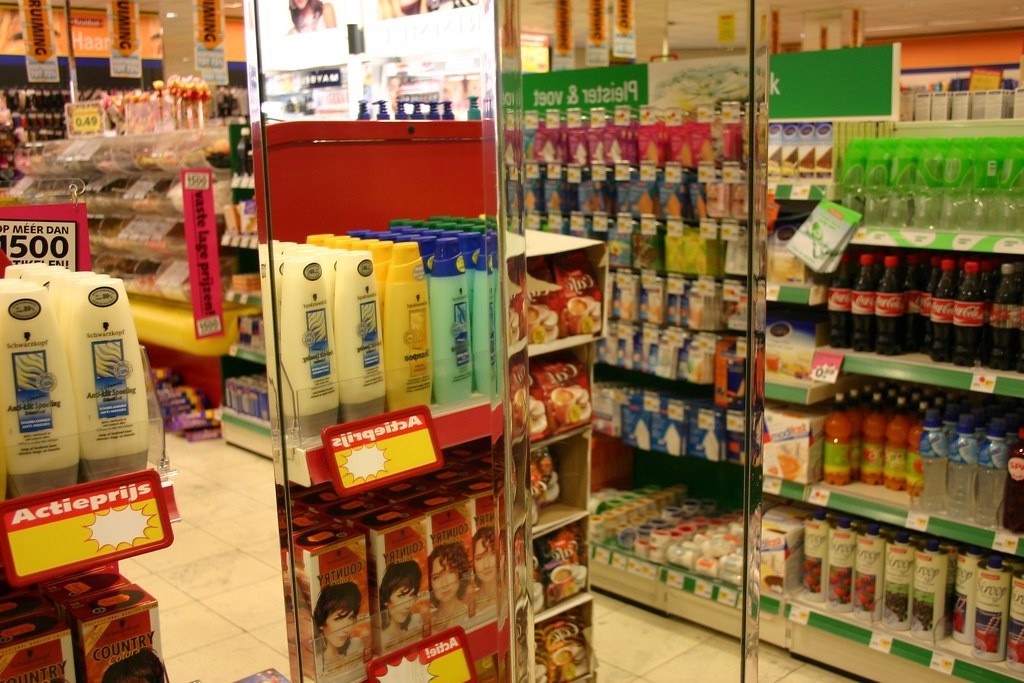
[287,0,336,35]
[313,526,498,664]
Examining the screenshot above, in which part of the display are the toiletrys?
[355,93,482,121]
[1,262,150,499]
[258,215,503,439]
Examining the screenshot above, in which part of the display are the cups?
[528,306,546,328]
[568,297,592,320]
[547,566,577,600]
[550,388,581,412]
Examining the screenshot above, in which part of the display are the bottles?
[827,248,1024,375]
[842,137,1023,232]
[824,382,1024,531]
[237,125,251,174]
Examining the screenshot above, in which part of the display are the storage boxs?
[494,0,759,683]
[290,443,499,671]
[525,230,606,683]
[0,561,165,683]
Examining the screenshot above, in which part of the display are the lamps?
[0,0,301,683]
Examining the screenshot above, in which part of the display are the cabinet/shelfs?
[0,178,183,683]
[760,111,1023,683]
[503,56,750,640]
[10,131,272,457]
